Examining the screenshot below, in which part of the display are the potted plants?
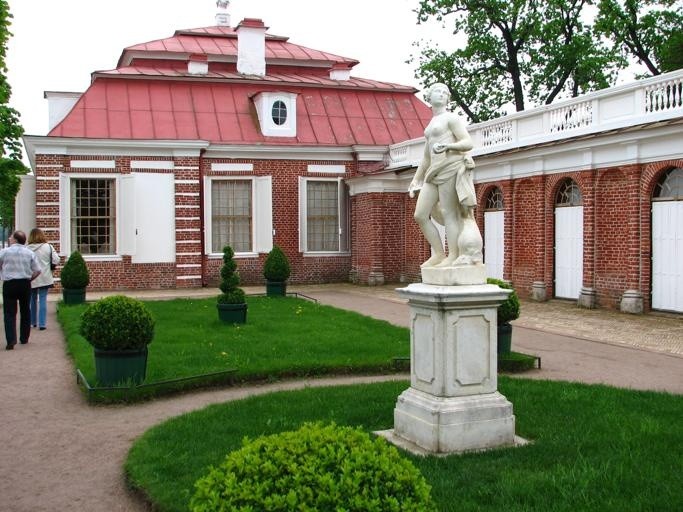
[216,245,248,324]
[262,247,291,298]
[60,252,89,306]
[76,296,156,389]
[486,277,521,357]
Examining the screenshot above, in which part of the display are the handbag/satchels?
[49,243,55,271]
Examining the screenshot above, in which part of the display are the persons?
[0,229,42,350]
[407,82,473,267]
[25,228,61,331]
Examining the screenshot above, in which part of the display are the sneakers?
[5,325,47,351]
